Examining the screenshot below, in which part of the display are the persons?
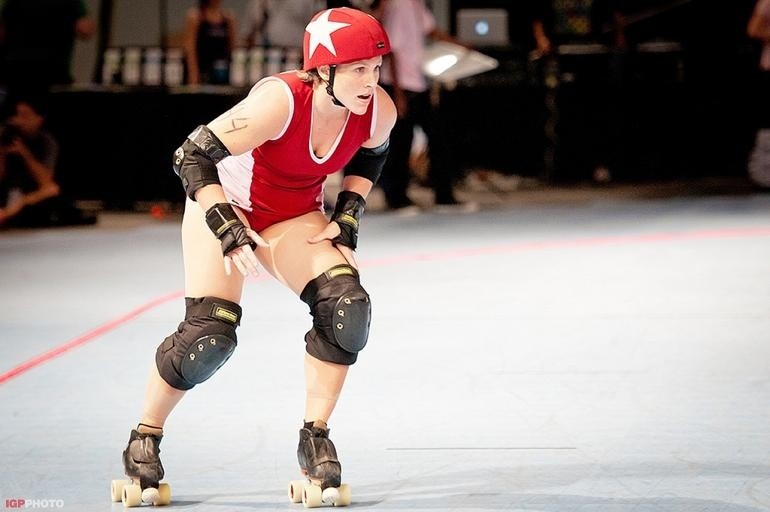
[532,1,617,182]
[186,1,235,86]
[121,6,398,482]
[747,1,770,187]
[1,0,97,234]
[325,0,482,216]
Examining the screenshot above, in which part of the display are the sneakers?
[110,423,170,508]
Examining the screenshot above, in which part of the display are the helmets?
[301,7,391,72]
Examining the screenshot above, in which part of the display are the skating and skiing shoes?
[288,420,350,508]
